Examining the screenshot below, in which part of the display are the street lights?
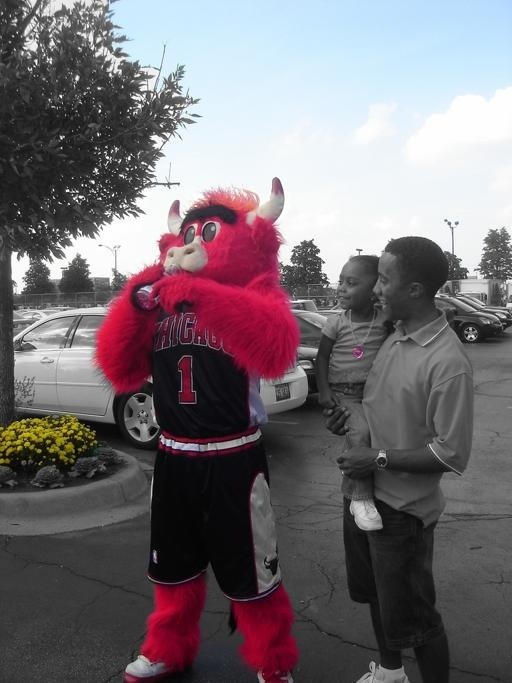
[97,242,121,278]
[444,218,460,254]
[354,247,364,256]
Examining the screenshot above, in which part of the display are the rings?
[340,471,345,478]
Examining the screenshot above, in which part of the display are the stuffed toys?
[91,177,301,683]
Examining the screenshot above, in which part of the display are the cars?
[294,344,323,399]
[290,292,512,346]
[12,306,76,341]
[11,304,309,446]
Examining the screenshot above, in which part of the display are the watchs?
[374,448,389,473]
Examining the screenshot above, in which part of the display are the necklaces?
[348,307,377,359]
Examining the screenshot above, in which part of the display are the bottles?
[134,259,184,311]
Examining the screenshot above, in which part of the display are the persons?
[316,256,396,532]
[322,237,476,683]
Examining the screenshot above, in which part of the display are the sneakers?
[348,496,384,532]
[355,661,411,683]
